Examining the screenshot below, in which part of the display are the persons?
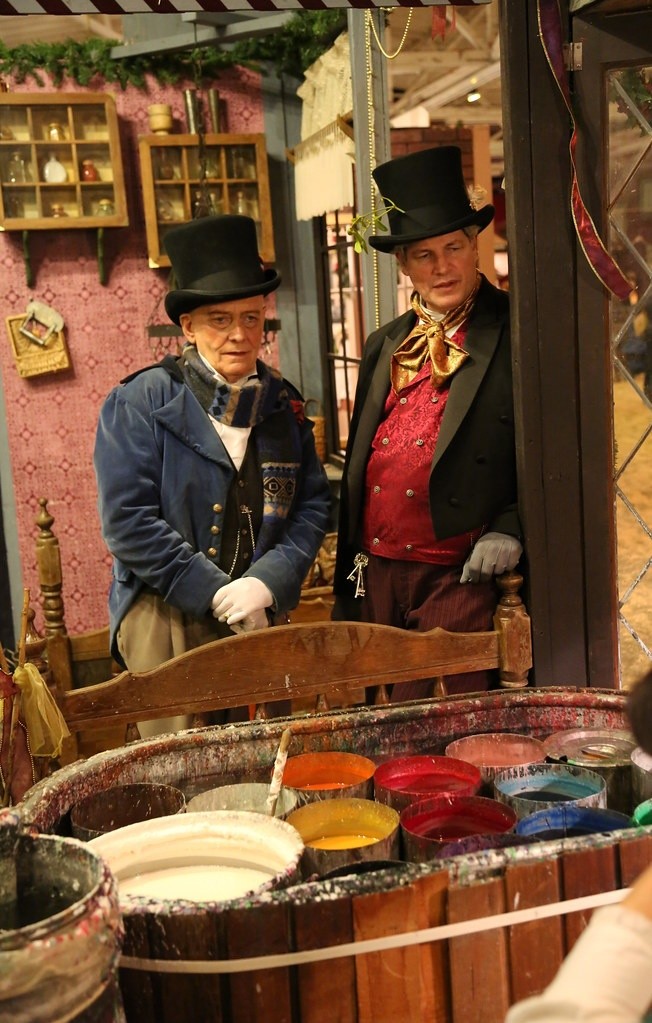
[505,663,652,1023]
[92,214,334,741]
[327,143,524,700]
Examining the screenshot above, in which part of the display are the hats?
[368,146,495,255]
[164,213,281,327]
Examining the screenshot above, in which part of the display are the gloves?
[230,608,268,633]
[459,531,523,584]
[211,576,273,625]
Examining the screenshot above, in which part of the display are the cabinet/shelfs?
[0,92,129,231]
[140,132,276,267]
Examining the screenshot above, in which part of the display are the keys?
[346,560,365,599]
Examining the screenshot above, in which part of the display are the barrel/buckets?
[0,831,126,1023]
[70,726,652,902]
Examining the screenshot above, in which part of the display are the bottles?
[233,191,249,215]
[81,159,96,181]
[42,149,66,183]
[192,192,209,218]
[7,151,27,183]
[156,147,173,180]
